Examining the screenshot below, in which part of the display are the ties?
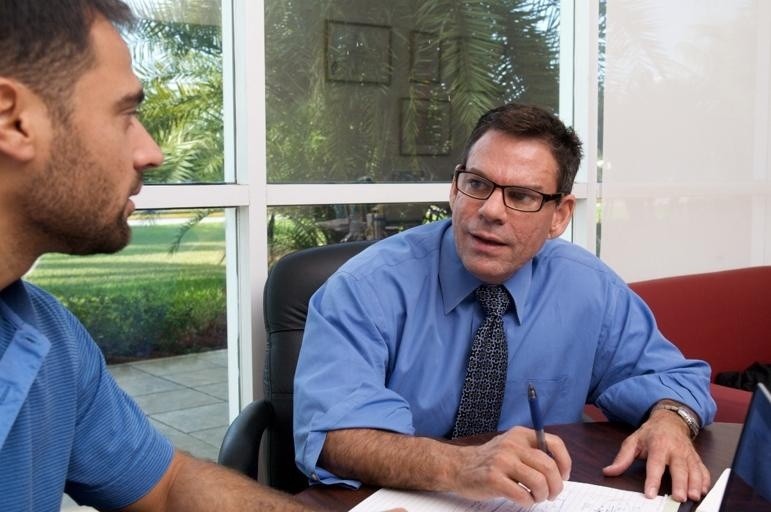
[453,285,512,439]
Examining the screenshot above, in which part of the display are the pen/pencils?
[527,382,549,454]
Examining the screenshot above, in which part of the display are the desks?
[291,422,770,512]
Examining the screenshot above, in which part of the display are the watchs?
[652,404,700,441]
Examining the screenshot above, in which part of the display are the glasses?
[454,165,568,212]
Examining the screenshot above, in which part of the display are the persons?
[0,0,409,512]
[293,104,718,507]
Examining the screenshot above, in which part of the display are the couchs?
[584,266,770,420]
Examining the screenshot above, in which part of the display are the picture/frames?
[320,16,512,158]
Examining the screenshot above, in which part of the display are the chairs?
[215,238,379,496]
